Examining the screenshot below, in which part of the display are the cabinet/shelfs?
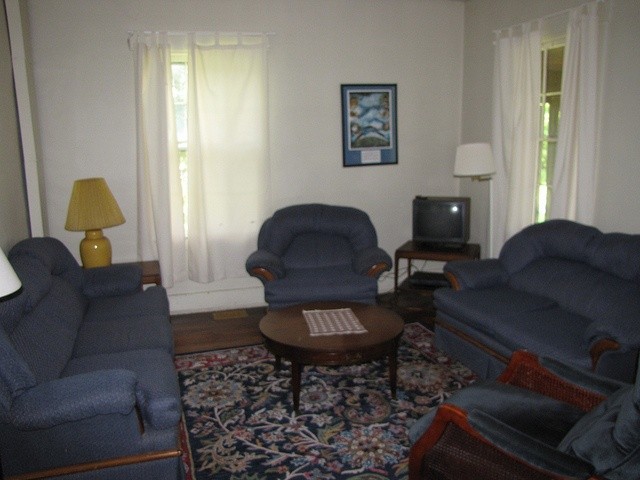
[394,240,480,328]
[109,262,163,290]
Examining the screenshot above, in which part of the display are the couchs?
[2,237,182,475]
[433,219,640,384]
[406,351,639,480]
[244,205,393,310]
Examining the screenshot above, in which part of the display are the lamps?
[63,178,128,269]
[451,142,496,183]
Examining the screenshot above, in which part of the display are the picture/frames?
[342,82,401,166]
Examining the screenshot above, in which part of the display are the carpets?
[173,318,484,479]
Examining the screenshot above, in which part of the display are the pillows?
[406,382,586,443]
